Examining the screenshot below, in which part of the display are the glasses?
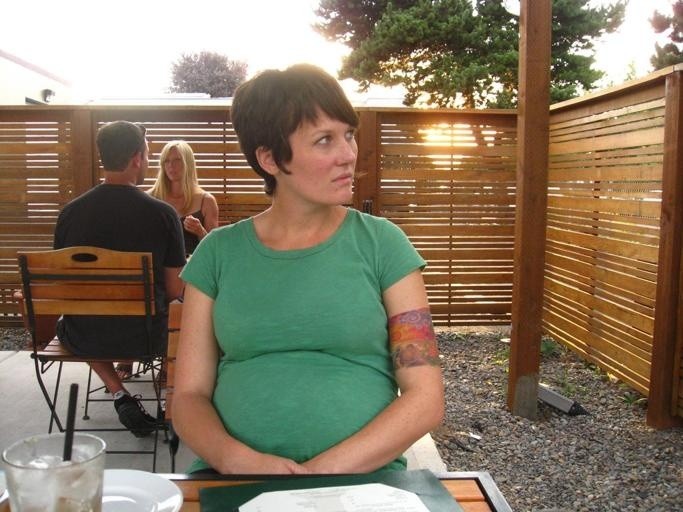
[131,125,146,157]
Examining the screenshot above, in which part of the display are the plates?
[101,468,184,512]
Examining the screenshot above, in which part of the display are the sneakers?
[115,393,154,438]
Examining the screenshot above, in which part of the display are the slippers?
[115,364,133,378]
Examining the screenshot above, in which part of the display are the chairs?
[16,242,178,473]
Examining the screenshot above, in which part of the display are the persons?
[112,139,220,390]
[170,64,446,477]
[52,121,187,439]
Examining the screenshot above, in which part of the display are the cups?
[1,431,107,512]
